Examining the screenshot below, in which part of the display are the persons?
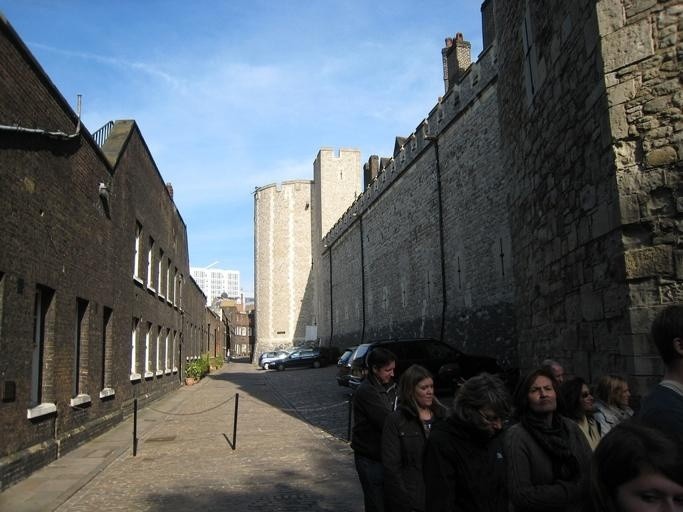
[620,303,683,431]
[349,346,513,512]
[502,359,638,511]
[586,428,683,512]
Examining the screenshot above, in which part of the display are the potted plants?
[183,360,200,386]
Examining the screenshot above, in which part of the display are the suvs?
[347,337,497,393]
[336,344,359,386]
[267,346,328,371]
[258,350,289,371]
